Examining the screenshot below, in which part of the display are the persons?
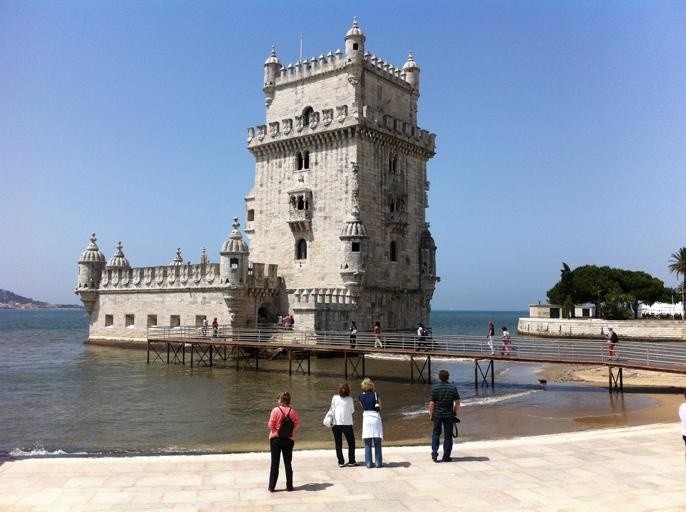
[601,327,620,361]
[568,309,572,319]
[415,322,429,352]
[357,377,384,469]
[201,315,208,337]
[500,326,512,356]
[266,390,300,492]
[211,317,219,338]
[486,320,496,356]
[258,311,295,330]
[374,321,384,350]
[428,368,461,463]
[678,389,686,465]
[328,380,358,468]
[348,321,358,349]
[642,311,683,320]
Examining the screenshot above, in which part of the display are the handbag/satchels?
[487,335,490,339]
[374,391,381,412]
[323,395,336,428]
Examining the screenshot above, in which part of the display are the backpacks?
[421,327,432,339]
[278,407,293,438]
[351,329,357,335]
[612,332,618,343]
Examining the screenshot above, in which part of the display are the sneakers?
[375,464,384,468]
[287,487,294,492]
[268,486,276,493]
[338,462,346,467]
[442,456,453,462]
[347,462,360,467]
[367,463,375,468]
[431,453,438,462]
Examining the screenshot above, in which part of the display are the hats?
[488,320,495,326]
[608,326,614,329]
